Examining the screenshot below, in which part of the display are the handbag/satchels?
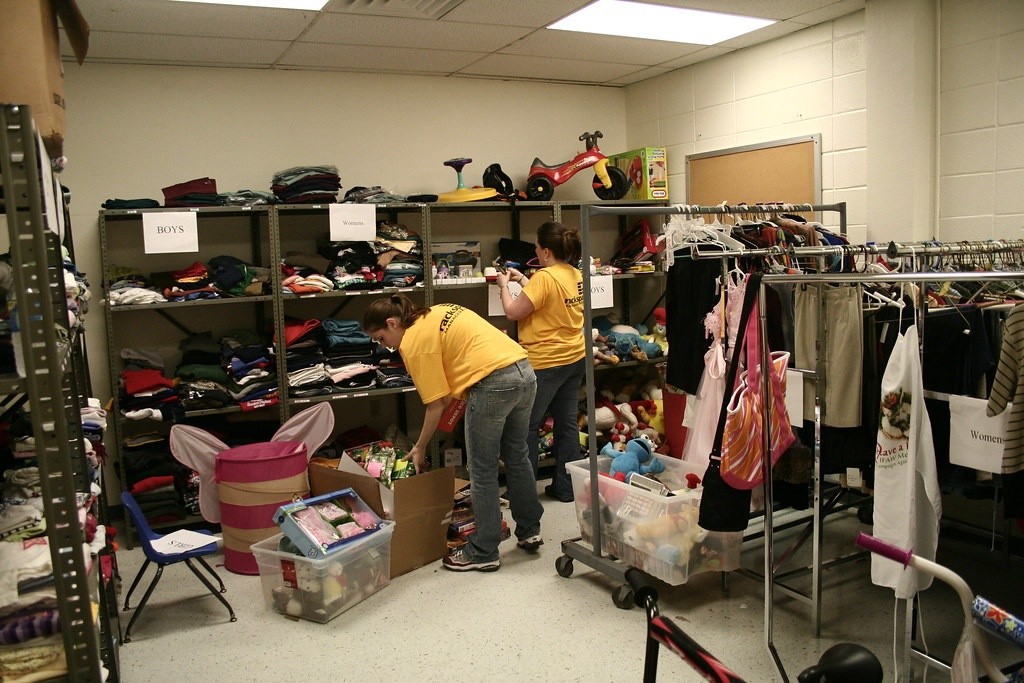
[719,275,796,490]
[698,453,752,532]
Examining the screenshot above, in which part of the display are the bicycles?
[623,567,885,683]
[853,529,1024,682]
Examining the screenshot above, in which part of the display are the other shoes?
[500,490,510,501]
[545,485,574,502]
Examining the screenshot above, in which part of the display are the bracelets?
[518,275,525,283]
[501,285,509,289]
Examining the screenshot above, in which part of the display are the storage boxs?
[606,147,668,199]
[272,487,387,562]
[0,0,90,159]
[565,453,743,587]
[309,458,455,581]
[431,240,481,273]
[250,520,396,624]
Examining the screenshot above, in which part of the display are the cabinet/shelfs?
[273,203,437,470]
[101,205,284,552]
[0,104,121,683]
[426,200,559,469]
[558,198,672,370]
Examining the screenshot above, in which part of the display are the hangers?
[655,201,1024,310]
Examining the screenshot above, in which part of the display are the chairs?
[120,494,236,645]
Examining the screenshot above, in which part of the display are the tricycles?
[525,130,629,201]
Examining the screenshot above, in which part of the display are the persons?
[496,222,586,503]
[363,291,538,572]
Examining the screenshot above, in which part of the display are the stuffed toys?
[538,379,665,460]
[585,434,703,547]
[591,308,669,366]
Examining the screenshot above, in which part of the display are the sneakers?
[517,533,544,548]
[442,549,501,572]
